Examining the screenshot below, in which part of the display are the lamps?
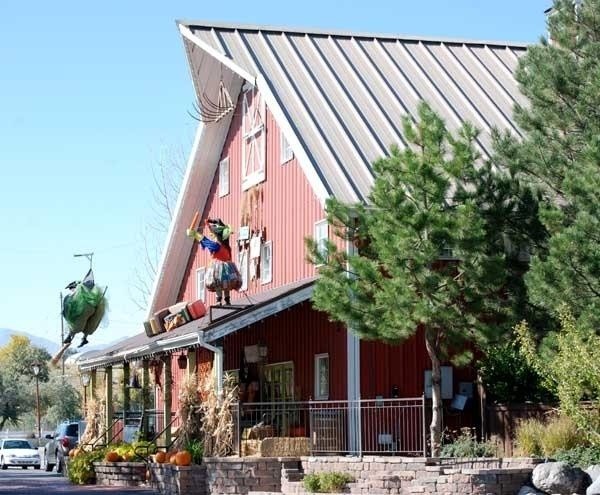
[178,350,189,369]
[81,371,91,387]
[257,336,268,358]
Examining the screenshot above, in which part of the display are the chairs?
[312,410,348,457]
[419,410,460,455]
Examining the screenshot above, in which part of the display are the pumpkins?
[294,427,305,436]
[74,448,81,456]
[155,451,192,465]
[106,452,119,462]
[68,449,74,456]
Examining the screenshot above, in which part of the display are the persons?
[239,372,264,416]
[233,379,243,409]
[185,217,240,306]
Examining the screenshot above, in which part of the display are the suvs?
[38,417,77,474]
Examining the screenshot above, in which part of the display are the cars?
[0,439,42,470]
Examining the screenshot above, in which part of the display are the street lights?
[30,358,44,446]
[79,371,90,419]
